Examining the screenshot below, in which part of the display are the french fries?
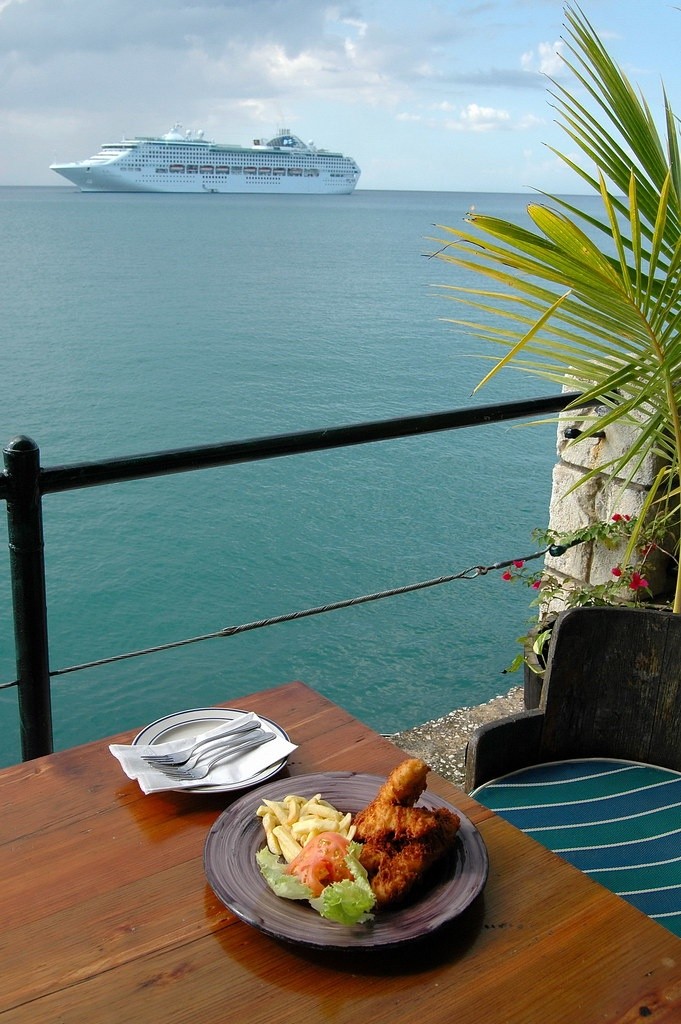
[255,792,356,863]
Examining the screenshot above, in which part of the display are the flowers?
[502,512,660,673]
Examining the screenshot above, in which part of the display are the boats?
[244,167,256,174]
[258,167,271,174]
[216,167,229,173]
[188,168,198,172]
[273,168,285,175]
[200,166,213,172]
[170,165,184,172]
[289,167,302,176]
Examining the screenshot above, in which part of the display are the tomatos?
[285,832,356,895]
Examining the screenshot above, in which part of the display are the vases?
[522,612,562,710]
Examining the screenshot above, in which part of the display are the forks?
[140,720,261,765]
[153,728,266,775]
[167,733,277,781]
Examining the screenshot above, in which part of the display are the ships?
[48,121,362,196]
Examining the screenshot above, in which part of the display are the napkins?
[109,711,298,794]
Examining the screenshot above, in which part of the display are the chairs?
[459,606,681,939]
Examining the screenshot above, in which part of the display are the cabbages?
[255,841,378,926]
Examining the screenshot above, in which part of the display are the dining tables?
[0,681,681,1024]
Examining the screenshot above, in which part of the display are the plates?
[202,771,489,950]
[131,707,290,793]
